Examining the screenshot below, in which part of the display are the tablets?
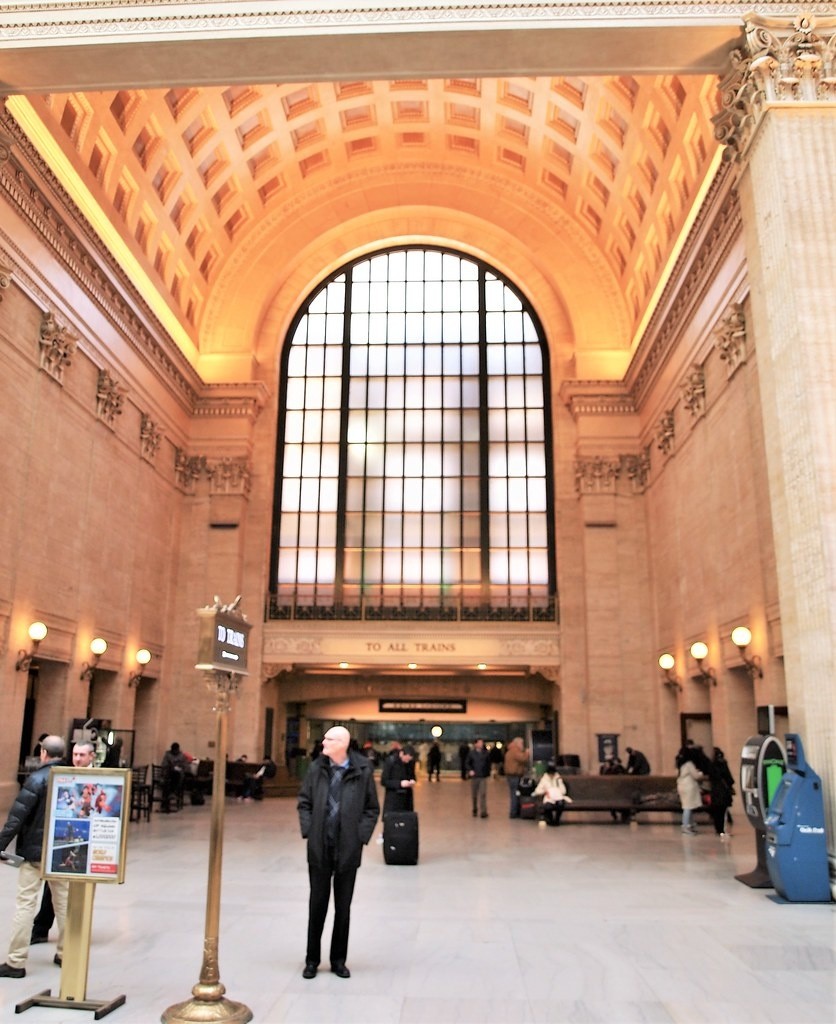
[2,852,26,868]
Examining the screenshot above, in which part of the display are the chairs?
[123,764,184,822]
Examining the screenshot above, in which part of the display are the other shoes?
[31,935,49,943]
[53,953,61,967]
[0,962,27,978]
[681,824,697,836]
[472,810,478,817]
[480,812,489,818]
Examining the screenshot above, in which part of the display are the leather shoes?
[303,960,321,979]
[331,963,351,978]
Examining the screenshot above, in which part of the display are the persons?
[673,738,736,839]
[597,747,651,822]
[0,736,124,978]
[310,735,570,827]
[296,725,380,979]
[159,742,276,814]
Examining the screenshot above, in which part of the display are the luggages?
[383,811,419,865]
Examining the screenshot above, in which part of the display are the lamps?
[16,622,47,670]
[129,649,151,688]
[658,654,683,694]
[731,627,763,679]
[79,638,107,679]
[691,641,717,687]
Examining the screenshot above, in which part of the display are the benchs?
[536,773,710,823]
[197,757,302,797]
